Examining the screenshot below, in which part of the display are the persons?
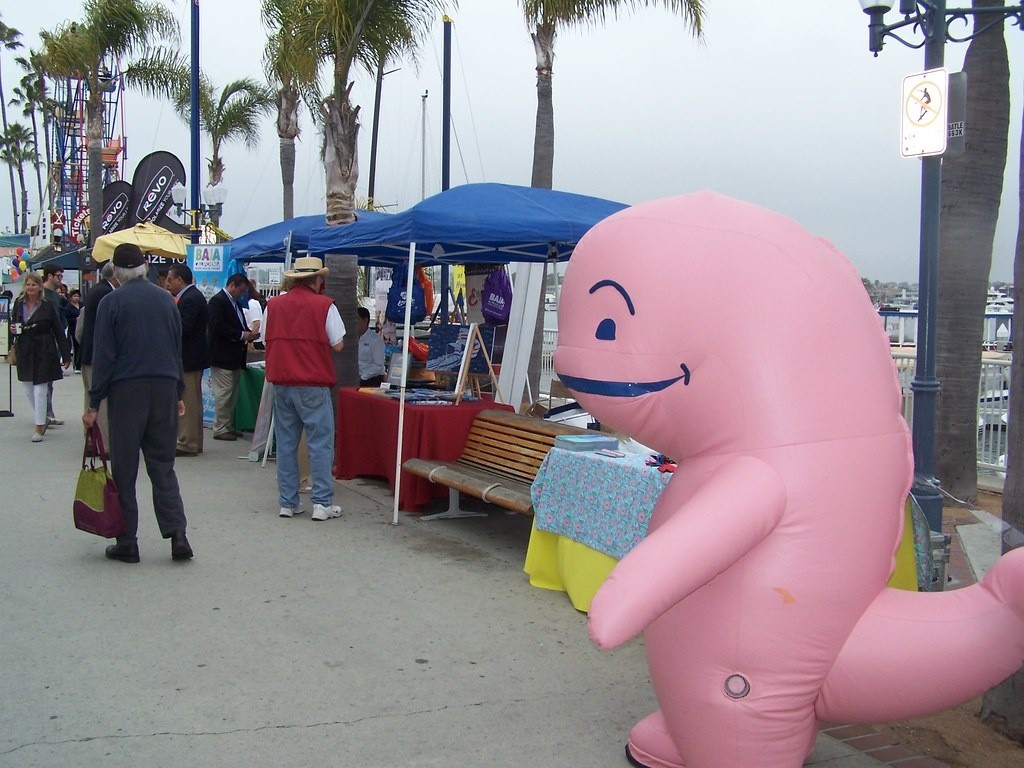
[90,66,112,82]
[261,257,346,521]
[50,207,66,245]
[167,263,211,457]
[357,307,386,388]
[82,242,194,563]
[155,271,179,303]
[41,263,69,424]
[242,278,267,350]
[0,285,24,360]
[207,274,260,441]
[55,282,85,375]
[82,261,120,459]
[10,271,72,442]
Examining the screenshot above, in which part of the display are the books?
[554,434,619,451]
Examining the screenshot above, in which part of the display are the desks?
[522,437,930,612]
[232,361,266,433]
[331,386,515,514]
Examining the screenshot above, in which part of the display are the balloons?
[11,247,29,280]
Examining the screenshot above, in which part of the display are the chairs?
[401,409,615,515]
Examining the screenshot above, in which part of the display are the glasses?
[167,278,177,283]
[54,274,64,279]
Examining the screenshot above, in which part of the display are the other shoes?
[105,543,140,563]
[216,430,244,440]
[172,533,193,560]
[75,365,82,374]
[175,449,202,457]
[32,417,51,442]
[49,418,66,425]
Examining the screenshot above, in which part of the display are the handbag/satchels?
[7,344,18,365]
[386,260,434,324]
[482,270,514,325]
[73,422,127,539]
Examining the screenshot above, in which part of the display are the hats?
[283,257,329,278]
[114,243,146,269]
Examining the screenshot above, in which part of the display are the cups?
[15,323,22,334]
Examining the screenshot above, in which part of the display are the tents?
[259,183,645,524]
[0,234,30,258]
[91,217,191,263]
[224,209,402,270]
[32,245,93,270]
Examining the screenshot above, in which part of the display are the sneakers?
[311,504,342,521]
[279,502,305,517]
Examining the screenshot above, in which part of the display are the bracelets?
[87,404,98,412]
